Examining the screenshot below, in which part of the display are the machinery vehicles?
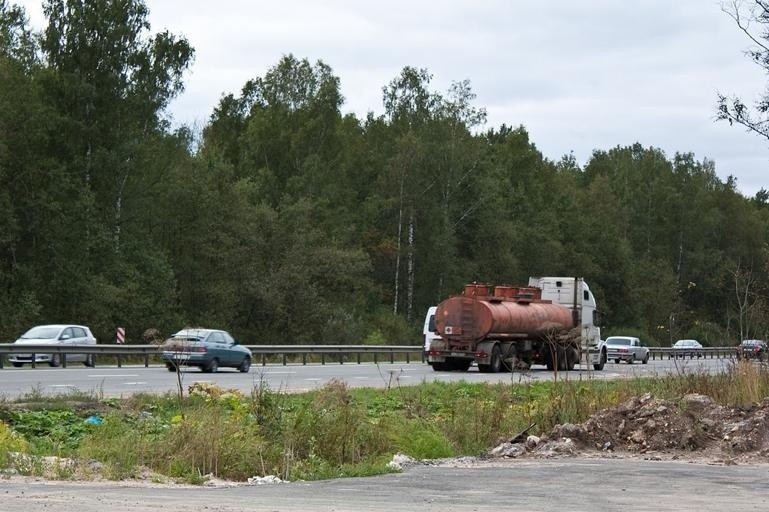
[428,276,608,373]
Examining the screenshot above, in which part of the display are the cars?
[10,325,98,368]
[604,336,650,364]
[669,339,704,357]
[737,340,769,362]
[160,327,253,375]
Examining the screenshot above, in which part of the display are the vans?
[422,306,443,355]
